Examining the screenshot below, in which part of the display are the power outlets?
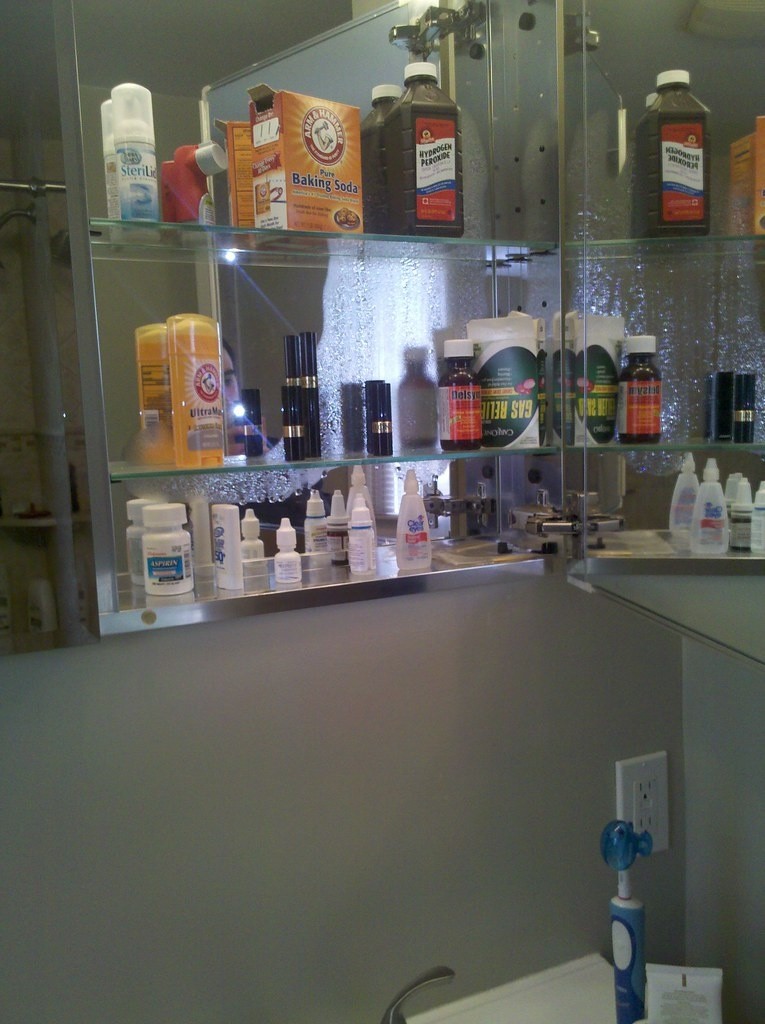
[616,750,670,852]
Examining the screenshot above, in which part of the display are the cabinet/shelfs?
[17,0,560,639]
[565,2,764,576]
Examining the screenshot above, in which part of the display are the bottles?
[439,337,483,452]
[126,498,149,585]
[665,452,765,555]
[275,517,302,583]
[345,465,376,524]
[139,501,197,597]
[627,65,710,241]
[358,84,402,234]
[618,333,662,444]
[382,61,468,238]
[303,488,328,555]
[239,509,265,560]
[326,489,377,575]
[396,469,433,569]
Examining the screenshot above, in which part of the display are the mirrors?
[550,2,765,664]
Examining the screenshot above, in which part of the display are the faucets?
[380,966,456,1024]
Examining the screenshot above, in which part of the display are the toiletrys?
[346,465,377,549]
[669,452,730,554]
[165,313,225,468]
[396,470,432,570]
[212,503,244,590]
[188,499,212,565]
[645,962,724,1024]
[134,322,174,464]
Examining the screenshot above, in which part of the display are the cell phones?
[232,401,245,442]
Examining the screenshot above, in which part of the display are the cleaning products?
[161,161,175,222]
[173,139,228,225]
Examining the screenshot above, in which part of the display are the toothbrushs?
[599,820,653,1023]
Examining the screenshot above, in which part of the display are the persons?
[124,341,306,561]
